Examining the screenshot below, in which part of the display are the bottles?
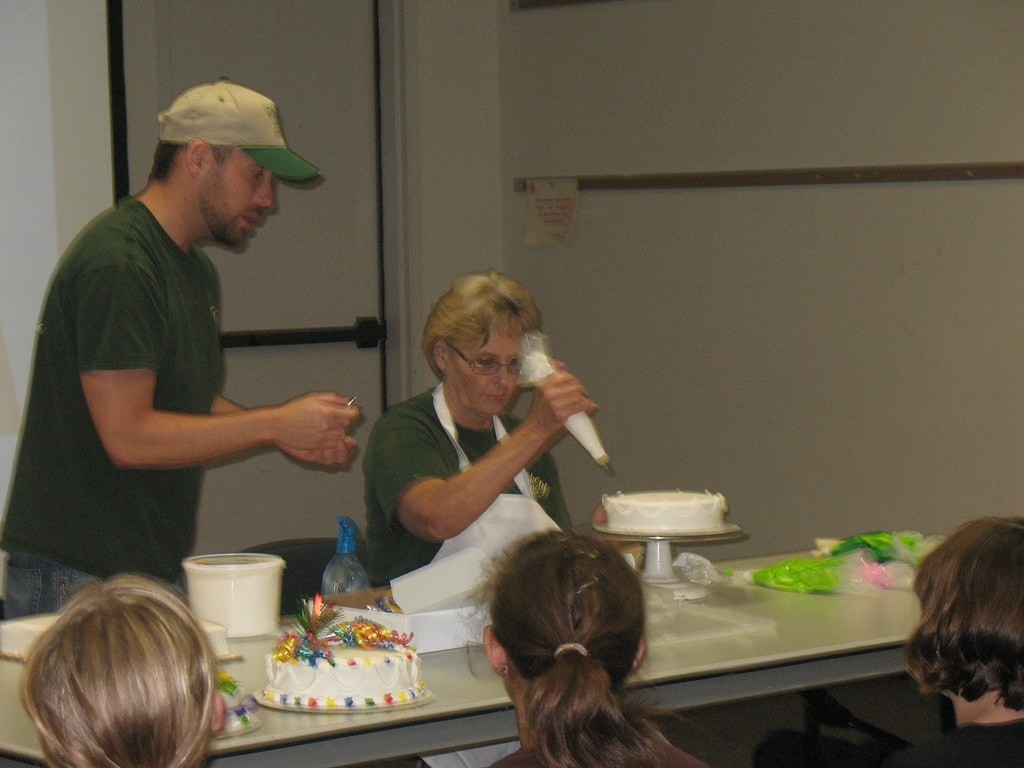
[745,549,877,594]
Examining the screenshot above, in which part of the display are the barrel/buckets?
[182,551,286,638]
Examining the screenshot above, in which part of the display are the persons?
[362,270,599,587]
[21,575,226,768]
[888,516,1024,768]
[0,76,365,621]
[484,530,709,768]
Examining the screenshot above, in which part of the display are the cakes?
[262,595,426,708]
[602,489,728,534]
[214,669,249,729]
[0,614,229,661]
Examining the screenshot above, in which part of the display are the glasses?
[445,340,523,377]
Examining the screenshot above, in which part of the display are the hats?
[158,79,320,185]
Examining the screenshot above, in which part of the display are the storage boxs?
[310,584,492,652]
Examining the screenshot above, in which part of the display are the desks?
[0,549,927,767]
